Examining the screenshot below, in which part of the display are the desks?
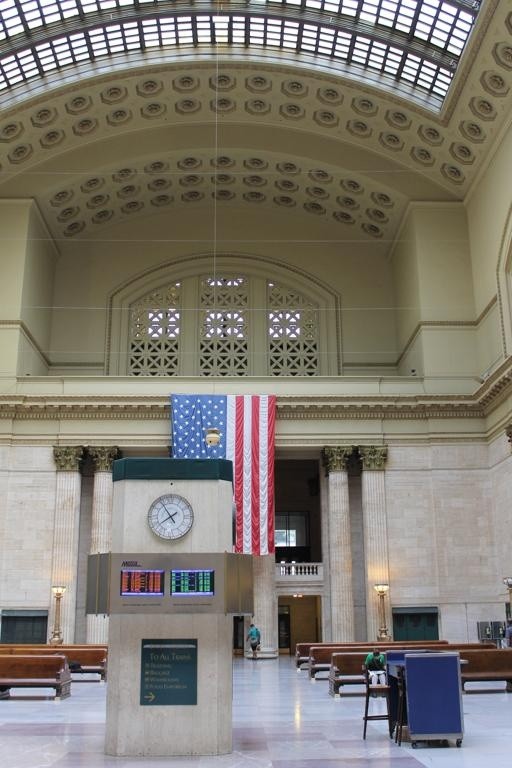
[386,650,465,748]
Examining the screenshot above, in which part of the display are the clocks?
[147,494,194,540]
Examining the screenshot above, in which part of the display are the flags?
[168,393,276,556]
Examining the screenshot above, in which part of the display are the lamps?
[49,586,67,644]
[374,584,393,641]
[503,577,512,617]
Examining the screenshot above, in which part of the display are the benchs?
[0,644,108,699]
[296,640,512,698]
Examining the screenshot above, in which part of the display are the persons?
[364,648,388,697]
[246,623,260,659]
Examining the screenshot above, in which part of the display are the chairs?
[361,665,405,746]
